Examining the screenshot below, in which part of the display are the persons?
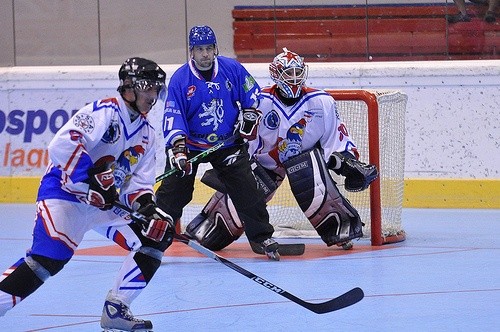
[0,56,174,332]
[183,50,379,251]
[152,26,281,261]
[445,0,500,22]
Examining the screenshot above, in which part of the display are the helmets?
[269,51,309,98]
[117,57,167,92]
[189,25,219,56]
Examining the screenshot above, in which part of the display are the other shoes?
[484,12,497,23]
[447,11,471,23]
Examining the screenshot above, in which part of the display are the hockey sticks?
[156,132,242,182]
[116,202,366,314]
[240,217,305,256]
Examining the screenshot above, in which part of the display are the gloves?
[233,107,263,140]
[136,200,174,243]
[168,144,193,177]
[86,161,120,210]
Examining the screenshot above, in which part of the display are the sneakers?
[260,237,280,261]
[101,288,154,331]
